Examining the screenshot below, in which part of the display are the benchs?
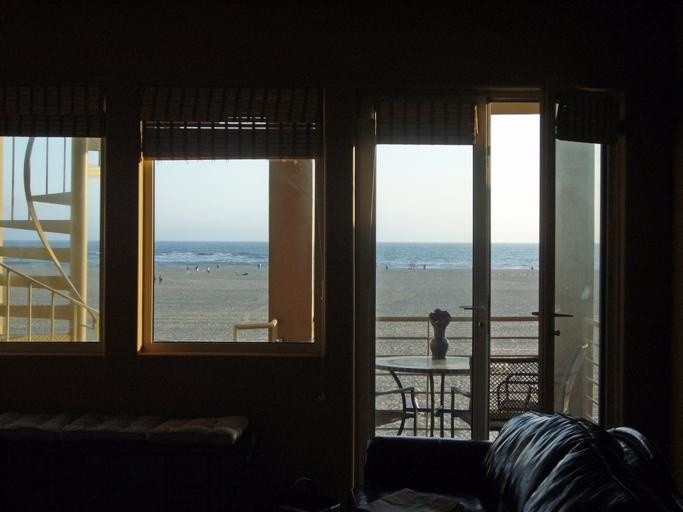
[0,412,258,510]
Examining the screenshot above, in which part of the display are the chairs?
[375,388,418,436]
[450,358,543,438]
[498,342,589,431]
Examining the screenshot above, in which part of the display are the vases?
[430,321,448,358]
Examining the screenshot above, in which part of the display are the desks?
[375,355,471,437]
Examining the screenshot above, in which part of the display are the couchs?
[340,410,682,510]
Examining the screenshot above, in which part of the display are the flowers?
[429,308,452,322]
